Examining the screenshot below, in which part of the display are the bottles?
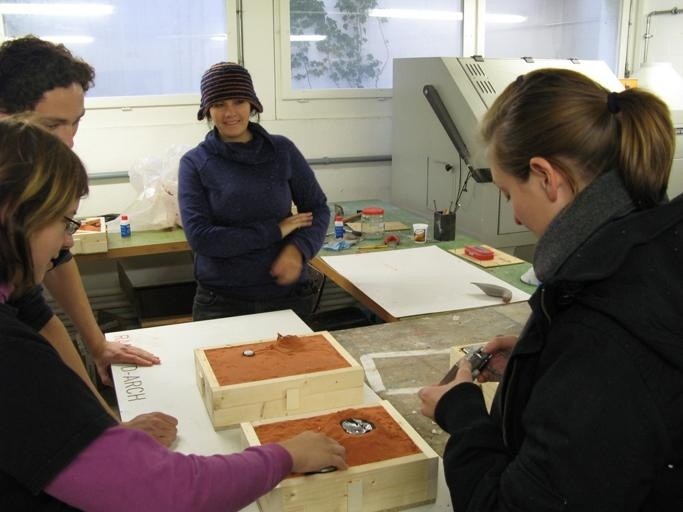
[359,207,387,246]
[120,215,132,240]
[334,216,343,240]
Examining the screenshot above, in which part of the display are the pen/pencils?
[358,245,389,249]
[433,200,453,215]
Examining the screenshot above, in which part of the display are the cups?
[433,211,457,242]
[412,223,429,244]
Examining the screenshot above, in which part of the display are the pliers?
[384,232,400,245]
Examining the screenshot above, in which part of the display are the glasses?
[61,214,83,237]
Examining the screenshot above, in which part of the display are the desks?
[308,199,541,321]
[68,215,193,260]
[330,300,534,458]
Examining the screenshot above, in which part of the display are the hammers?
[344,210,362,223]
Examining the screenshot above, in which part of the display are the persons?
[420,67,682,511]
[0,35,178,450]
[0,114,347,512]
[177,62,331,324]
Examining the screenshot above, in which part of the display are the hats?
[196,60,261,122]
